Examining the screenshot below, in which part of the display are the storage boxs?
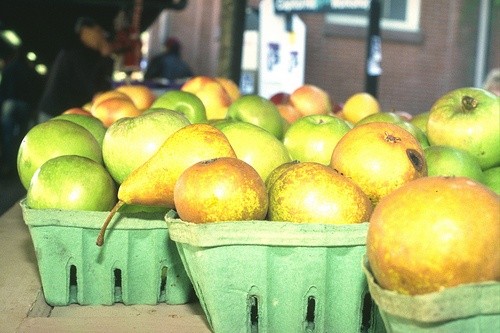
[20,197,500,333]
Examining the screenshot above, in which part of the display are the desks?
[0,199,211,333]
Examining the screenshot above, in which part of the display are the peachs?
[64,75,377,126]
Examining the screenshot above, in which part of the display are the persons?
[36,17,115,125]
[145,38,192,89]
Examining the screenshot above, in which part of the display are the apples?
[16,87,500,213]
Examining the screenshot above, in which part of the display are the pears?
[95,124,499,297]
